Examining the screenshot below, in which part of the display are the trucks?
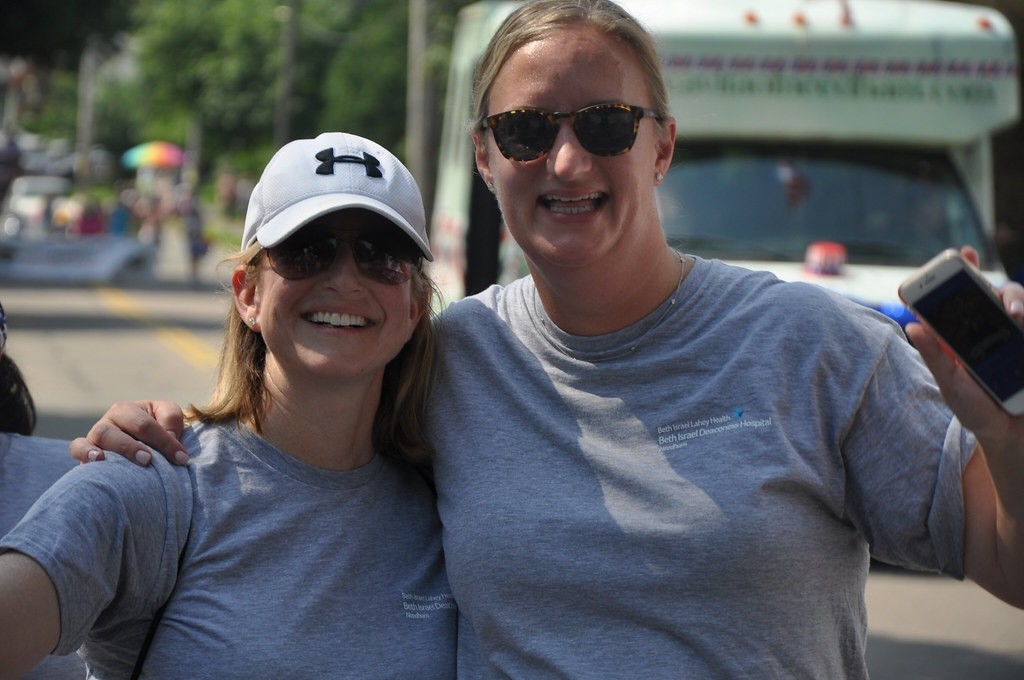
[430,2,1024,335]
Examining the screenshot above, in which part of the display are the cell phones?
[898,247,1024,418]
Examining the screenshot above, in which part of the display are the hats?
[241,131,434,262]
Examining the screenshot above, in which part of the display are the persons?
[0,131,468,677]
[71,0,1024,679]
[38,165,249,288]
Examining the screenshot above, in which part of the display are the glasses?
[265,223,424,285]
[478,101,662,161]
